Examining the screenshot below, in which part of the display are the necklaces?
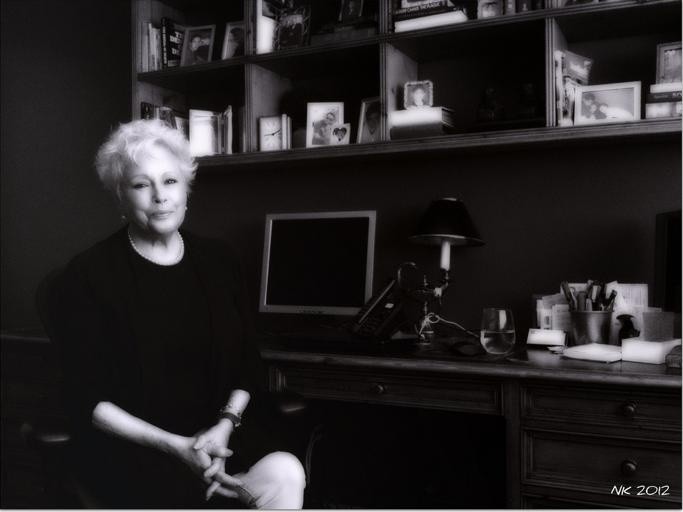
[126,226,185,267]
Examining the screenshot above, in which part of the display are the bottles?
[479,306,516,355]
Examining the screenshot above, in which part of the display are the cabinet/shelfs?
[128,0,682,168]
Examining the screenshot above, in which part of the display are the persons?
[45,117,312,510]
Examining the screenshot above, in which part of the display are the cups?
[569,308,614,346]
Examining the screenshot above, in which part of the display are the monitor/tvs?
[258,210,377,336]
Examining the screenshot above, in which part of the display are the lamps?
[397,192,481,341]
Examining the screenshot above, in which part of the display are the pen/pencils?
[561,280,618,312]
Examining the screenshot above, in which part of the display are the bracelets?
[220,409,240,429]
[219,402,243,417]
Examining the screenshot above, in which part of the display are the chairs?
[20,260,310,508]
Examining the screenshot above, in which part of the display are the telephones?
[351,279,407,337]
